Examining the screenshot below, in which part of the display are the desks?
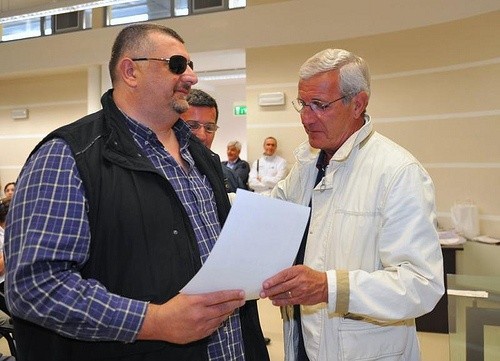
[415,243,464,334]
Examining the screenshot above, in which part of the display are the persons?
[180,49,444,361]
[4,24,288,361]
[0,183,17,361]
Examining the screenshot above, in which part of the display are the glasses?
[291,93,353,112]
[188,121,220,134]
[132,55,194,75]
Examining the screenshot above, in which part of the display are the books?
[437,230,467,245]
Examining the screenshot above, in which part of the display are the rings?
[288,291,293,298]
